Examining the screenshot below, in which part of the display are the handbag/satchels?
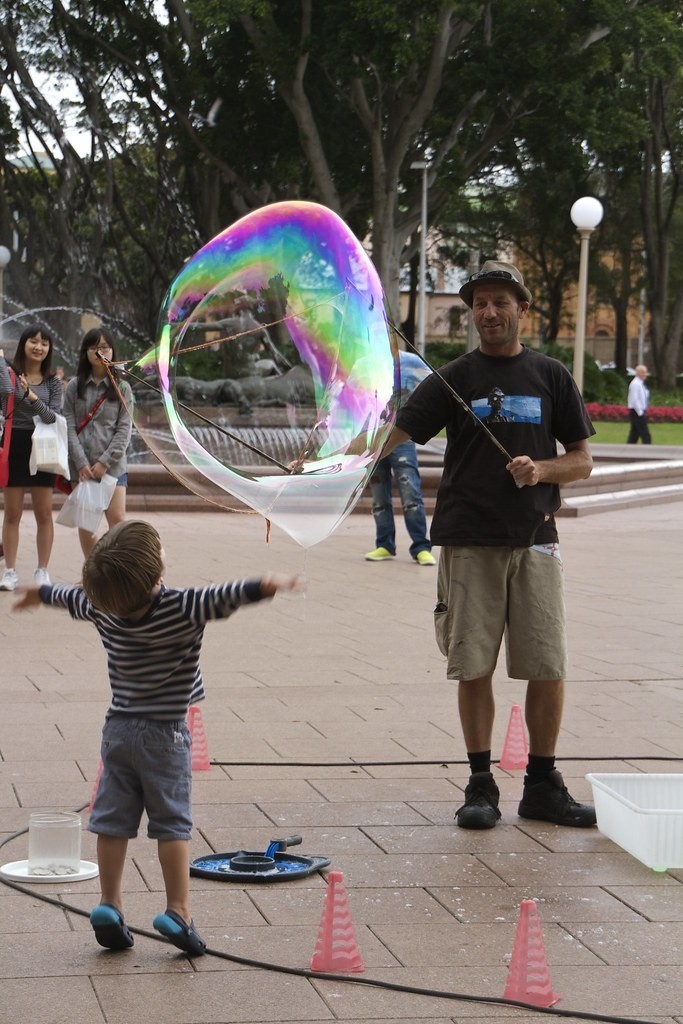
[0,410,5,441]
[0,448,8,487]
[30,412,71,480]
[55,475,72,495]
[55,474,117,533]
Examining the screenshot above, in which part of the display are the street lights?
[566,195,605,403]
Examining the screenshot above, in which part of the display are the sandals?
[153,909,206,954]
[90,904,134,948]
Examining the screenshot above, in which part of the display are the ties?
[642,384,647,404]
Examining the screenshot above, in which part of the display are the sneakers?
[454,772,502,827]
[34,568,52,588]
[518,770,597,826]
[417,551,435,565]
[365,547,395,560]
[0,568,18,591]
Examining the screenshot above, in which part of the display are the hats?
[459,260,532,309]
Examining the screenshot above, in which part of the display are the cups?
[29,811,82,874]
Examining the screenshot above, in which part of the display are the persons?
[624,364,652,445]
[0,320,133,592]
[349,323,437,566]
[10,520,307,954]
[168,294,294,379]
[282,260,596,827]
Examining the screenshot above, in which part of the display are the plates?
[0,860,99,883]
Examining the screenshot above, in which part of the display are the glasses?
[88,344,112,352]
